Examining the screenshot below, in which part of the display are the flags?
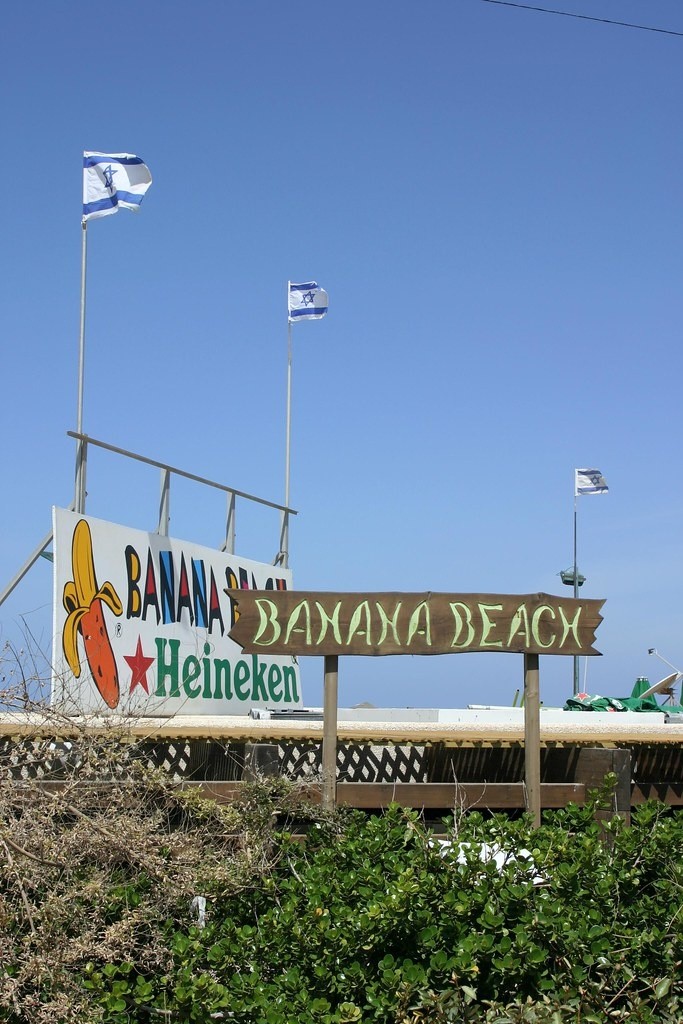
[288,280,331,322]
[575,467,610,496]
[81,150,153,224]
